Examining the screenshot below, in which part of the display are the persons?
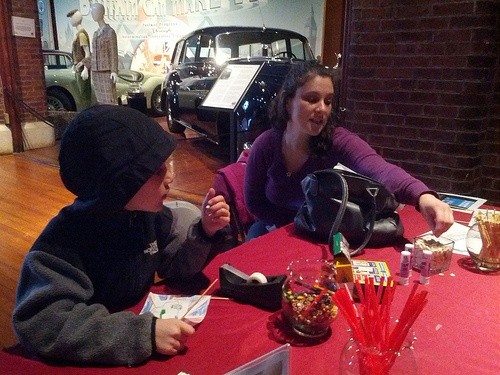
[67,9,91,109]
[11,104,230,367]
[91,3,118,104]
[243,61,454,242]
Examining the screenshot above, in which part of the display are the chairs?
[214,161,252,240]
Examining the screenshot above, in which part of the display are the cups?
[411,231,454,276]
[283,258,339,339]
[466,220,500,272]
[339,313,419,375]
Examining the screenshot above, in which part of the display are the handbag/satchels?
[293,169,405,247]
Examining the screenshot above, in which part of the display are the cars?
[42,49,167,118]
[163,25,321,157]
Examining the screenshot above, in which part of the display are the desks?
[0,198,500,375]
